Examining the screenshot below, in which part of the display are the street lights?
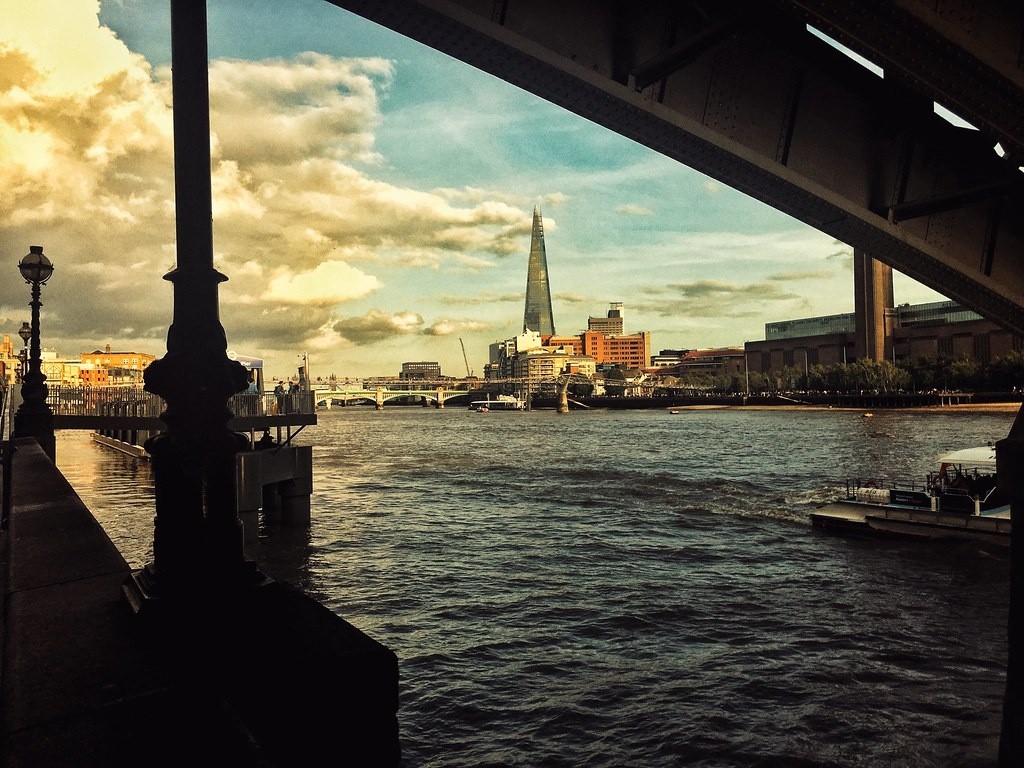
[12,244,57,438]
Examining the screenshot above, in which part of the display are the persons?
[285,382,297,414]
[274,381,285,415]
[248,379,258,413]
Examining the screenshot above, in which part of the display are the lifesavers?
[864,482,879,489]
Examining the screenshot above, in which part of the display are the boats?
[808,440,1011,550]
[471,396,529,411]
